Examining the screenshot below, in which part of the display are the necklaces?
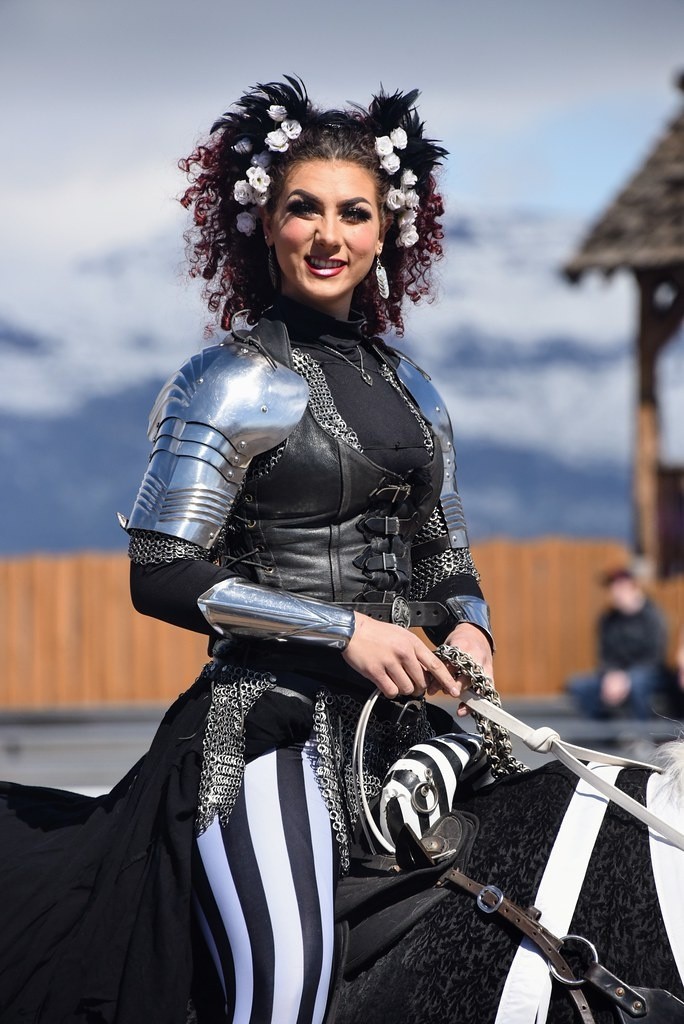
[323,335,376,390]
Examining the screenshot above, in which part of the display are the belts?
[333,596,450,629]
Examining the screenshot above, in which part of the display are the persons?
[556,567,679,722]
[123,73,492,1024]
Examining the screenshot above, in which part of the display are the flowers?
[230,104,303,239]
[375,127,421,250]
